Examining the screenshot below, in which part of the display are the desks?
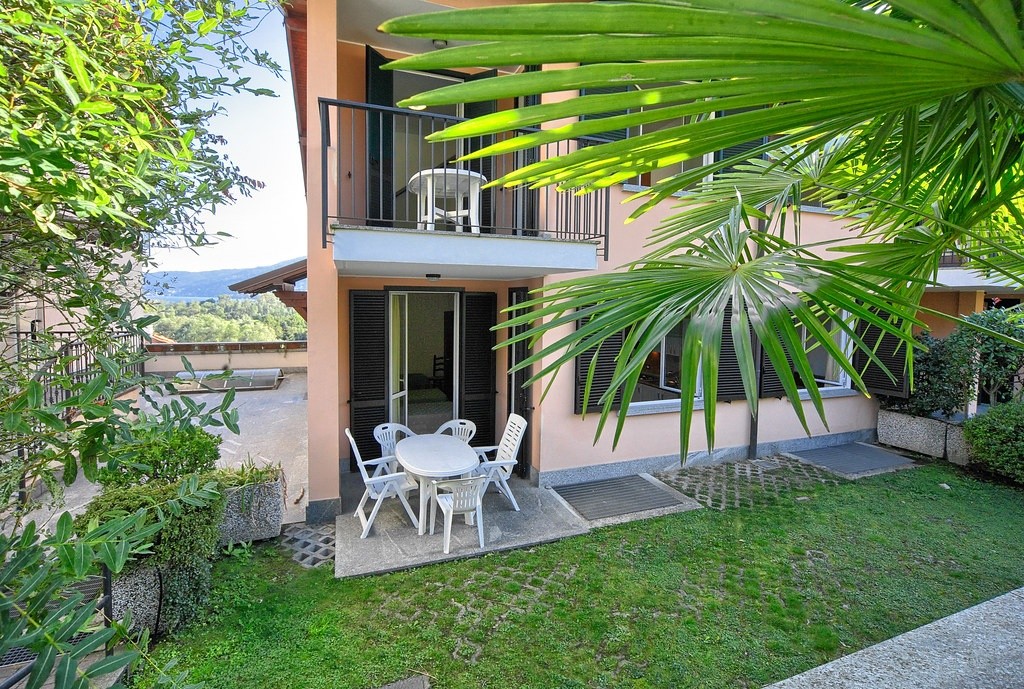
[405,164,487,235]
[393,433,481,537]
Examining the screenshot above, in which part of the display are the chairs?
[428,354,446,390]
[345,410,548,555]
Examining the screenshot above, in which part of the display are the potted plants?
[74,451,288,640]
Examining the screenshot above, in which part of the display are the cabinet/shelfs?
[634,383,678,402]
